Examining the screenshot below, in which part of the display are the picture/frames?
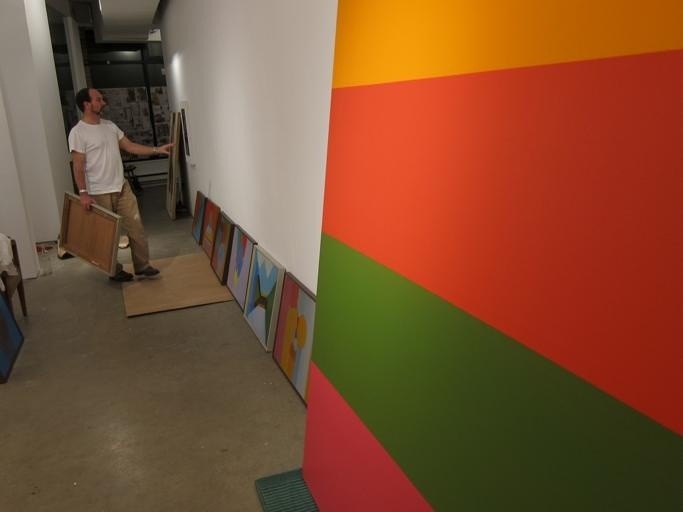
[190,191,316,408]
[59,192,123,278]
[0,292,24,383]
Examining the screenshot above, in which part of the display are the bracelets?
[79,190,88,193]
[153,147,157,154]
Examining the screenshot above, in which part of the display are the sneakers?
[108,270,134,284]
[133,266,160,276]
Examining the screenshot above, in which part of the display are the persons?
[68,88,177,283]
